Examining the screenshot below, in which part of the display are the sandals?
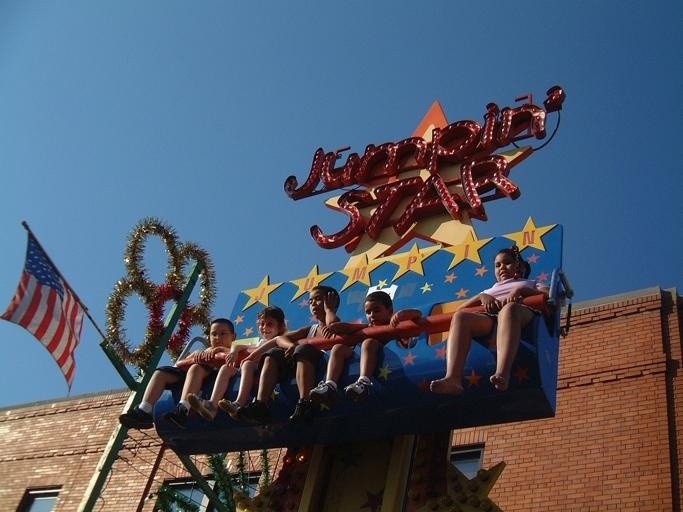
[220,397,243,421]
[186,393,219,422]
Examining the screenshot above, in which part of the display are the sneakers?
[309,380,338,403]
[290,399,315,421]
[117,404,154,430]
[164,404,188,431]
[238,399,269,425]
[344,376,375,401]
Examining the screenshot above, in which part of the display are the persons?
[235,285,340,424]
[429,245,549,395]
[187,305,286,422]
[116,319,237,430]
[309,291,422,402]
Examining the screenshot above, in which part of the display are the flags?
[1,234,86,398]
